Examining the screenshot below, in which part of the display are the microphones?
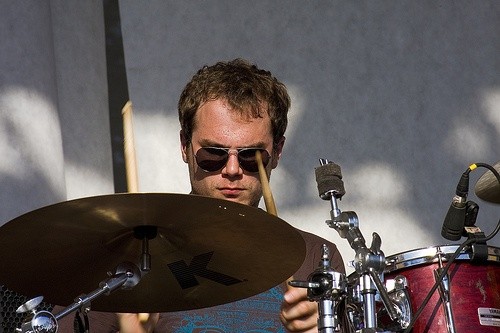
[440,171,470,241]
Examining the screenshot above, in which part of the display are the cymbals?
[0,190,307,313]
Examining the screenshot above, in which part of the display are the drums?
[349,245,500,333]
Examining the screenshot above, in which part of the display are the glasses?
[189,136,273,173]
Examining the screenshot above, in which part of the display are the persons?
[51,58,346,333]
